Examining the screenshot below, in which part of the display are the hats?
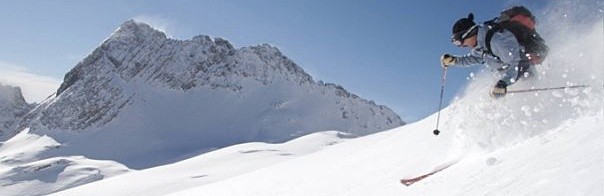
[453,14,478,39]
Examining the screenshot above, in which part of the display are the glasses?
[452,25,478,47]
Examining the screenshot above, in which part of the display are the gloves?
[492,80,506,98]
[441,54,457,68]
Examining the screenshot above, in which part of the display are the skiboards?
[400,155,463,188]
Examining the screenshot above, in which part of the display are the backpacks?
[484,6,547,64]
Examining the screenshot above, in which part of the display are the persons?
[440,12,534,101]
